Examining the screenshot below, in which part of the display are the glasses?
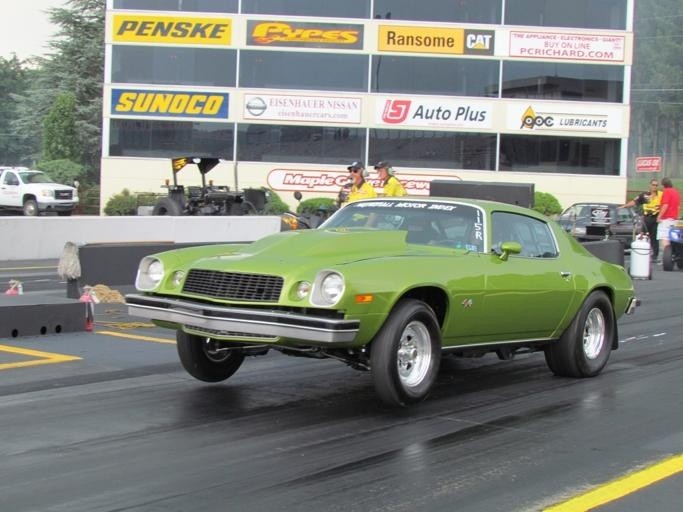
[349,170,358,173]
[652,184,658,186]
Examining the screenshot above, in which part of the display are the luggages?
[630,240,652,280]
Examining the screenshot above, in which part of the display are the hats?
[375,161,391,169]
[348,162,364,170]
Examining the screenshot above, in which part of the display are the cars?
[0,165,79,217]
[124,196,637,411]
[554,202,644,252]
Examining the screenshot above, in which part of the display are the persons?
[335,160,376,228]
[374,158,407,197]
[616,177,662,261]
[654,177,680,266]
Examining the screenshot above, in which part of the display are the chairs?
[399,216,441,245]
